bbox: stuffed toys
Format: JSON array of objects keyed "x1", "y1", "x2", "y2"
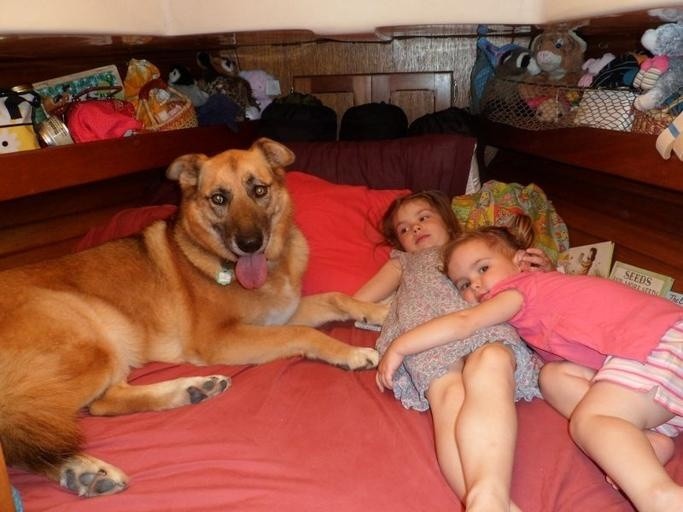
[{"x1": 479, "y1": 4, "x2": 683, "y2": 131}]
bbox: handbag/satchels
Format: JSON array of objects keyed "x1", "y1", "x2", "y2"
[
  {"x1": 0, "y1": 89, "x2": 42, "y2": 154},
  {"x1": 66, "y1": 95, "x2": 143, "y2": 143}
]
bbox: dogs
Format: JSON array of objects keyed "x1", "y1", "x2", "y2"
[{"x1": 0, "y1": 136, "x2": 394, "y2": 498}]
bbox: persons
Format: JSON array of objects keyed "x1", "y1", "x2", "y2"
[
  {"x1": 575, "y1": 248, "x2": 597, "y2": 275},
  {"x1": 372, "y1": 212, "x2": 683, "y2": 511},
  {"x1": 344, "y1": 187, "x2": 557, "y2": 510}
]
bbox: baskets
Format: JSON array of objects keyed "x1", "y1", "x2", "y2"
[{"x1": 133, "y1": 75, "x2": 198, "y2": 133}]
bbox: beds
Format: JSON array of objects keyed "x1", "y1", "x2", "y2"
[{"x1": 3, "y1": 134, "x2": 682, "y2": 512}]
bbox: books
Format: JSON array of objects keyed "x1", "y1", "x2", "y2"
[
  {"x1": 609, "y1": 260, "x2": 675, "y2": 300},
  {"x1": 564, "y1": 241, "x2": 614, "y2": 278}
]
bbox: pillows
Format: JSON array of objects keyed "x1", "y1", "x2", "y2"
[{"x1": 271, "y1": 169, "x2": 411, "y2": 257}]
